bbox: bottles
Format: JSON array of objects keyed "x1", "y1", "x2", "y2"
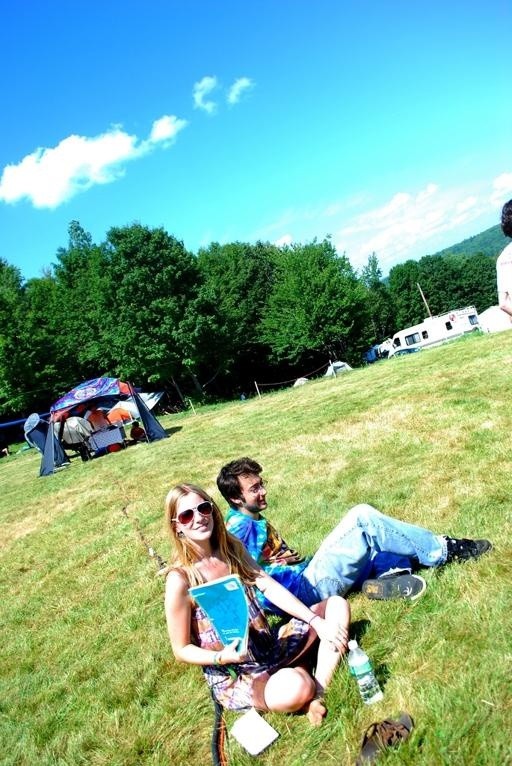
[{"x1": 345, "y1": 638, "x2": 384, "y2": 707}]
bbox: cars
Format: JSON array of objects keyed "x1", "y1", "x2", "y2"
[{"x1": 385, "y1": 347, "x2": 419, "y2": 360}]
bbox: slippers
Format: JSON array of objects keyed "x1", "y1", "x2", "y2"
[{"x1": 353, "y1": 712, "x2": 414, "y2": 766}]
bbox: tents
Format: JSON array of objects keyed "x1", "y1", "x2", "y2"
[
  {"x1": 322, "y1": 360, "x2": 355, "y2": 378},
  {"x1": 365, "y1": 344, "x2": 387, "y2": 363},
  {"x1": 23, "y1": 376, "x2": 170, "y2": 476},
  {"x1": 293, "y1": 377, "x2": 309, "y2": 388}
]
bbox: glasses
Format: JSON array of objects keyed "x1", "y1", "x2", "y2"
[
  {"x1": 241, "y1": 480, "x2": 268, "y2": 493},
  {"x1": 171, "y1": 501, "x2": 213, "y2": 526}
]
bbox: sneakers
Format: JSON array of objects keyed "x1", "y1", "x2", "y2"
[
  {"x1": 361, "y1": 573, "x2": 426, "y2": 601},
  {"x1": 438, "y1": 536, "x2": 491, "y2": 568}
]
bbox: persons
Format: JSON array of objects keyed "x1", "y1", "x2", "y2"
[
  {"x1": 494, "y1": 199, "x2": 512, "y2": 323},
  {"x1": 214, "y1": 456, "x2": 493, "y2": 615},
  {"x1": 130, "y1": 422, "x2": 146, "y2": 442},
  {"x1": 160, "y1": 483, "x2": 353, "y2": 729},
  {"x1": 86, "y1": 403, "x2": 113, "y2": 431}
]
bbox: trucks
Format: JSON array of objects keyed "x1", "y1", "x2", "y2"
[{"x1": 387, "y1": 303, "x2": 483, "y2": 361}]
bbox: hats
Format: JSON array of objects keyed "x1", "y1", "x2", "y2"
[{"x1": 281, "y1": 625, "x2": 318, "y2": 667}]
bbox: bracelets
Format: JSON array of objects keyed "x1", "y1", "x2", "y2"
[
  {"x1": 213, "y1": 652, "x2": 223, "y2": 670},
  {"x1": 307, "y1": 615, "x2": 319, "y2": 626}
]
